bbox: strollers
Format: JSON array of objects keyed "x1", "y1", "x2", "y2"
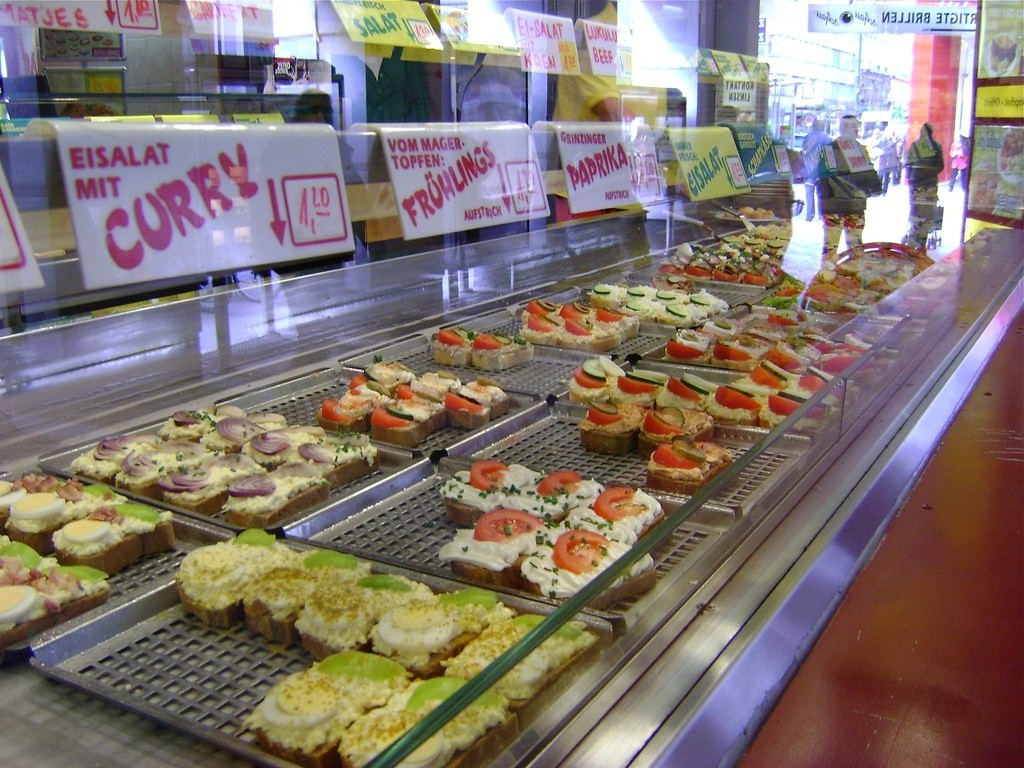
[{"x1": 901, "y1": 194, "x2": 944, "y2": 248}]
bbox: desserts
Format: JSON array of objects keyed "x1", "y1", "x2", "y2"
[{"x1": 0, "y1": 212, "x2": 935, "y2": 768}]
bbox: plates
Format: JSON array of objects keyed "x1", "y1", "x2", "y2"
[{"x1": 715, "y1": 214, "x2": 779, "y2": 225}]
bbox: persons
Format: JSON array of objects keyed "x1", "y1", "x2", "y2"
[
  {"x1": 255, "y1": 88, "x2": 386, "y2": 371},
  {"x1": 948, "y1": 134, "x2": 970, "y2": 192},
  {"x1": 907, "y1": 122, "x2": 945, "y2": 247},
  {"x1": 802, "y1": 117, "x2": 834, "y2": 222},
  {"x1": 817, "y1": 115, "x2": 882, "y2": 267},
  {"x1": 546, "y1": 0, "x2": 669, "y2": 292},
  {"x1": 864, "y1": 130, "x2": 905, "y2": 195}
]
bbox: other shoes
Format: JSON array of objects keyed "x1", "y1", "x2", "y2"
[{"x1": 806, "y1": 213, "x2": 814, "y2": 221}]
